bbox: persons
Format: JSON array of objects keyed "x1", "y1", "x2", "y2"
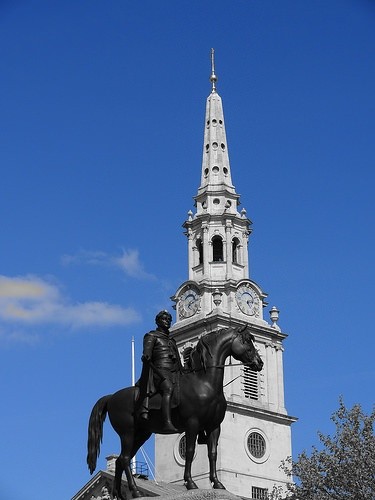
[{"x1": 136, "y1": 308, "x2": 183, "y2": 434}]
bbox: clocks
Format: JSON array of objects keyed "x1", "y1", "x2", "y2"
[
  {"x1": 178, "y1": 286, "x2": 199, "y2": 318},
  {"x1": 235, "y1": 285, "x2": 260, "y2": 315}
]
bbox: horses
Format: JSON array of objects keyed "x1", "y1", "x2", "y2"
[{"x1": 86, "y1": 322, "x2": 265, "y2": 500}]
